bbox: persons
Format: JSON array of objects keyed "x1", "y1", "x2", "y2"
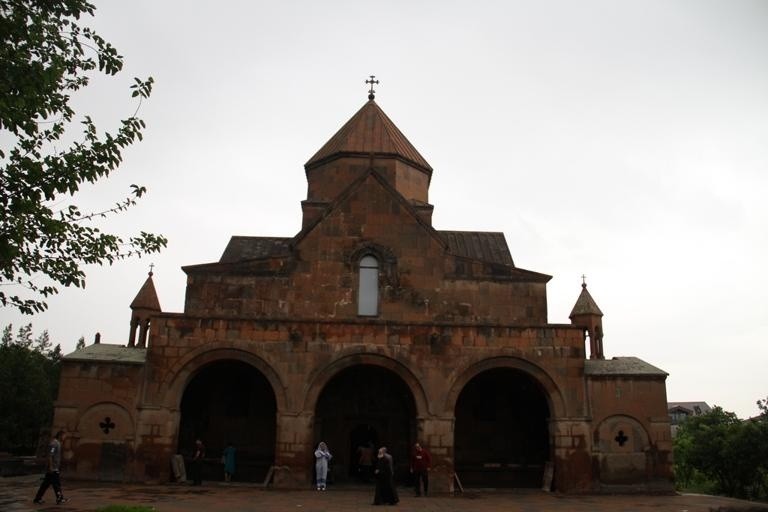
[
  {"x1": 381, "y1": 446, "x2": 399, "y2": 503},
  {"x1": 33, "y1": 430, "x2": 73, "y2": 505},
  {"x1": 188, "y1": 436, "x2": 207, "y2": 487},
  {"x1": 219, "y1": 442, "x2": 238, "y2": 482},
  {"x1": 370, "y1": 447, "x2": 395, "y2": 505},
  {"x1": 409, "y1": 441, "x2": 433, "y2": 498},
  {"x1": 314, "y1": 441, "x2": 334, "y2": 491},
  {"x1": 359, "y1": 441, "x2": 374, "y2": 476}
]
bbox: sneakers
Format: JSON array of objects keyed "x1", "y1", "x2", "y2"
[
  {"x1": 317, "y1": 486, "x2": 326, "y2": 492},
  {"x1": 56, "y1": 495, "x2": 72, "y2": 504},
  {"x1": 33, "y1": 499, "x2": 45, "y2": 504}
]
